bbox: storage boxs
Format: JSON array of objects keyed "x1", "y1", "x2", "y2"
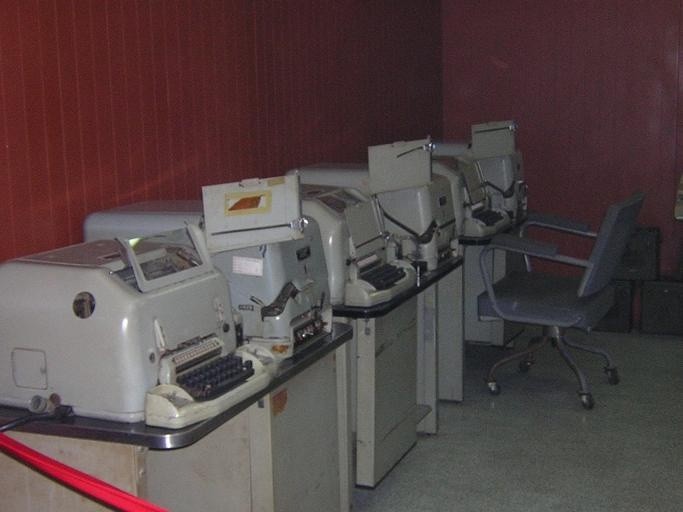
[
  {"x1": 593, "y1": 278, "x2": 632, "y2": 332},
  {"x1": 640, "y1": 275, "x2": 683, "y2": 336},
  {"x1": 610, "y1": 226, "x2": 660, "y2": 281}
]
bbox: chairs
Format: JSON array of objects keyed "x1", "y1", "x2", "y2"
[{"x1": 476, "y1": 192, "x2": 644, "y2": 410}]
